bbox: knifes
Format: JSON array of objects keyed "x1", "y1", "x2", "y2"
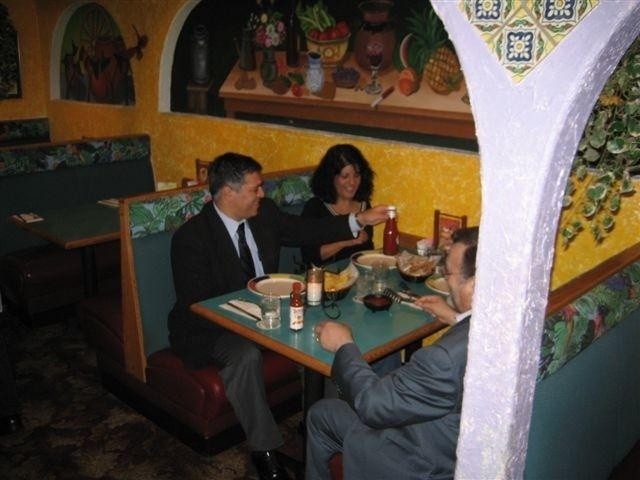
[
  {"x1": 371, "y1": 87, "x2": 395, "y2": 109},
  {"x1": 227, "y1": 302, "x2": 262, "y2": 323}
]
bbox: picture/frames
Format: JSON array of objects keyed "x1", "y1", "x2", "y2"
[{"x1": 1, "y1": 30, "x2": 23, "y2": 101}]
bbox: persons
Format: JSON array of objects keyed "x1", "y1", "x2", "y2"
[
  {"x1": 166, "y1": 152, "x2": 405, "y2": 478},
  {"x1": 306, "y1": 225, "x2": 478, "y2": 479},
  {"x1": 300, "y1": 143, "x2": 401, "y2": 376}
]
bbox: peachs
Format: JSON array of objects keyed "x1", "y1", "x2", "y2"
[{"x1": 398, "y1": 66, "x2": 421, "y2": 97}]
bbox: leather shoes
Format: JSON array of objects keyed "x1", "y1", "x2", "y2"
[
  {"x1": 251, "y1": 450, "x2": 289, "y2": 480},
  {"x1": 1, "y1": 415, "x2": 22, "y2": 436}
]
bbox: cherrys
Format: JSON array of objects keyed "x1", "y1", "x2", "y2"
[
  {"x1": 292, "y1": 83, "x2": 305, "y2": 98},
  {"x1": 233, "y1": 69, "x2": 257, "y2": 90}
]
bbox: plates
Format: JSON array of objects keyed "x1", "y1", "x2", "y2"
[
  {"x1": 349, "y1": 250, "x2": 398, "y2": 272},
  {"x1": 425, "y1": 273, "x2": 453, "y2": 296},
  {"x1": 247, "y1": 272, "x2": 310, "y2": 298}
]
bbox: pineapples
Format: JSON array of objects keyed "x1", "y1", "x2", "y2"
[{"x1": 403, "y1": 7, "x2": 464, "y2": 96}]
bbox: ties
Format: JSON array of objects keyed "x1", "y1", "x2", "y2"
[{"x1": 237, "y1": 223, "x2": 255, "y2": 278}]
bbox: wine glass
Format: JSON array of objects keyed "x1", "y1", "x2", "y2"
[{"x1": 363, "y1": 43, "x2": 384, "y2": 95}]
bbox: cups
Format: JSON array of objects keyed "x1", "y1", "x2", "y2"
[
  {"x1": 371, "y1": 261, "x2": 389, "y2": 294},
  {"x1": 356, "y1": 270, "x2": 377, "y2": 302},
  {"x1": 260, "y1": 294, "x2": 281, "y2": 329}
]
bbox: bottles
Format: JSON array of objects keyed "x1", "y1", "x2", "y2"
[
  {"x1": 288, "y1": 282, "x2": 304, "y2": 331},
  {"x1": 382, "y1": 205, "x2": 399, "y2": 255},
  {"x1": 286, "y1": 7, "x2": 301, "y2": 68}
]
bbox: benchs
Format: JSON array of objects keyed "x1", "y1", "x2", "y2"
[
  {"x1": 1, "y1": 118, "x2": 53, "y2": 145},
  {"x1": 118, "y1": 163, "x2": 419, "y2": 457},
  {"x1": 398, "y1": 244, "x2": 637, "y2": 480},
  {"x1": 2, "y1": 134, "x2": 156, "y2": 305}
]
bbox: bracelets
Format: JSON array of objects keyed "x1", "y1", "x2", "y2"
[{"x1": 354, "y1": 213, "x2": 365, "y2": 229}]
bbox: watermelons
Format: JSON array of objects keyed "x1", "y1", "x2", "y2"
[{"x1": 393, "y1": 31, "x2": 423, "y2": 81}]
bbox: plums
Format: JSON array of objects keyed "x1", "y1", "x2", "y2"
[{"x1": 271, "y1": 73, "x2": 291, "y2": 95}]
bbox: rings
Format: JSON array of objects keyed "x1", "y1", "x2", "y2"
[{"x1": 316, "y1": 336, "x2": 320, "y2": 342}]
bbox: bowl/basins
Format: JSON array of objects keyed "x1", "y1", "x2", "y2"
[{"x1": 399, "y1": 265, "x2": 436, "y2": 283}]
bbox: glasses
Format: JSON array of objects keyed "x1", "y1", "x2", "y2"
[{"x1": 438, "y1": 269, "x2": 454, "y2": 278}]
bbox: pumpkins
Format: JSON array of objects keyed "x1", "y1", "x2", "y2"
[{"x1": 88, "y1": 30, "x2": 127, "y2": 102}]
